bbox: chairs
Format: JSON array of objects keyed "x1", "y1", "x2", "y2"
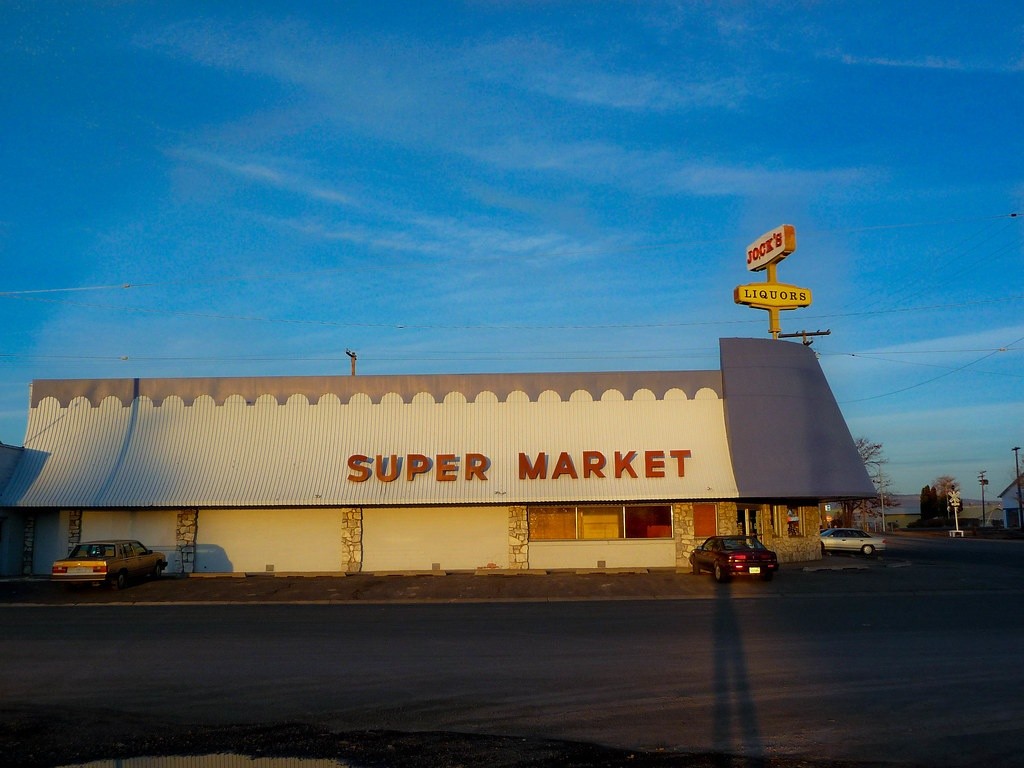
[
  {"x1": 77, "y1": 550, "x2": 88, "y2": 558},
  {"x1": 105, "y1": 550, "x2": 114, "y2": 556}
]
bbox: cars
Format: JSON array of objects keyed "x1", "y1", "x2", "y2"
[
  {"x1": 50, "y1": 539, "x2": 168, "y2": 590},
  {"x1": 688, "y1": 535, "x2": 780, "y2": 583},
  {"x1": 819, "y1": 528, "x2": 887, "y2": 554}
]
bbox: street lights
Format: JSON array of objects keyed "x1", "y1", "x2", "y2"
[{"x1": 1011, "y1": 446, "x2": 1024, "y2": 531}]
